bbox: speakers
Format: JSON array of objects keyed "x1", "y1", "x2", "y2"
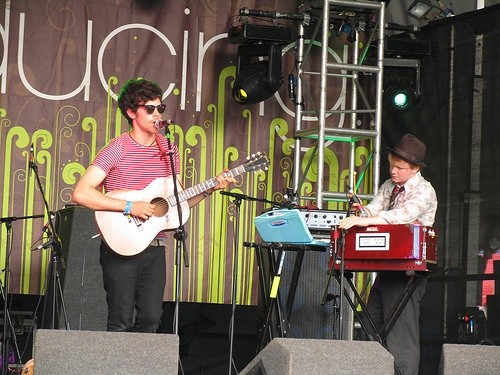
[
  {"x1": 438, "y1": 343, "x2": 500, "y2": 375},
  {"x1": 273, "y1": 243, "x2": 355, "y2": 340},
  {"x1": 44, "y1": 206, "x2": 109, "y2": 331},
  {"x1": 33, "y1": 329, "x2": 179, "y2": 375},
  {"x1": 238, "y1": 338, "x2": 394, "y2": 375}
]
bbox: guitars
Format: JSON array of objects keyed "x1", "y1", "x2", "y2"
[{"x1": 94, "y1": 151, "x2": 270, "y2": 257}]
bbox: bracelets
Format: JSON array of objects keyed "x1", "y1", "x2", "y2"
[{"x1": 123, "y1": 200, "x2": 132, "y2": 215}]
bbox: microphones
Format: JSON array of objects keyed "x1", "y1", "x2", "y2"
[
  {"x1": 37, "y1": 212, "x2": 56, "y2": 240},
  {"x1": 155, "y1": 119, "x2": 174, "y2": 130},
  {"x1": 29, "y1": 143, "x2": 34, "y2": 168},
  {"x1": 347, "y1": 185, "x2": 368, "y2": 218},
  {"x1": 38, "y1": 238, "x2": 55, "y2": 250},
  {"x1": 326, "y1": 294, "x2": 340, "y2": 301}
]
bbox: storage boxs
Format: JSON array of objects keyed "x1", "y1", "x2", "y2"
[{"x1": 331, "y1": 224, "x2": 438, "y2": 264}]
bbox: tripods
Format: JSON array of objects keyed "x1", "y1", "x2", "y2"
[{"x1": 32, "y1": 165, "x2": 70, "y2": 329}]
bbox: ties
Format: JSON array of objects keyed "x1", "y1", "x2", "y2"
[{"x1": 388, "y1": 185, "x2": 405, "y2": 210}]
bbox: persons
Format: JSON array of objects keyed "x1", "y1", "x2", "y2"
[
  {"x1": 338, "y1": 133, "x2": 437, "y2": 375},
  {"x1": 72, "y1": 79, "x2": 232, "y2": 333}
]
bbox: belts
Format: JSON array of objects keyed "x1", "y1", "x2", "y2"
[{"x1": 150, "y1": 240, "x2": 168, "y2": 248}]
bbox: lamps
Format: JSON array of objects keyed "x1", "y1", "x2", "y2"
[
  {"x1": 232, "y1": 58, "x2": 282, "y2": 105},
  {"x1": 407, "y1": 0, "x2": 432, "y2": 22},
  {"x1": 382, "y1": 78, "x2": 414, "y2": 113}
]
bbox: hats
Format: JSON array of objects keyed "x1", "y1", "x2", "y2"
[{"x1": 385, "y1": 134, "x2": 428, "y2": 168}]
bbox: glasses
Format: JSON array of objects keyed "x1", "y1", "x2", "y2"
[{"x1": 137, "y1": 104, "x2": 166, "y2": 114}]
